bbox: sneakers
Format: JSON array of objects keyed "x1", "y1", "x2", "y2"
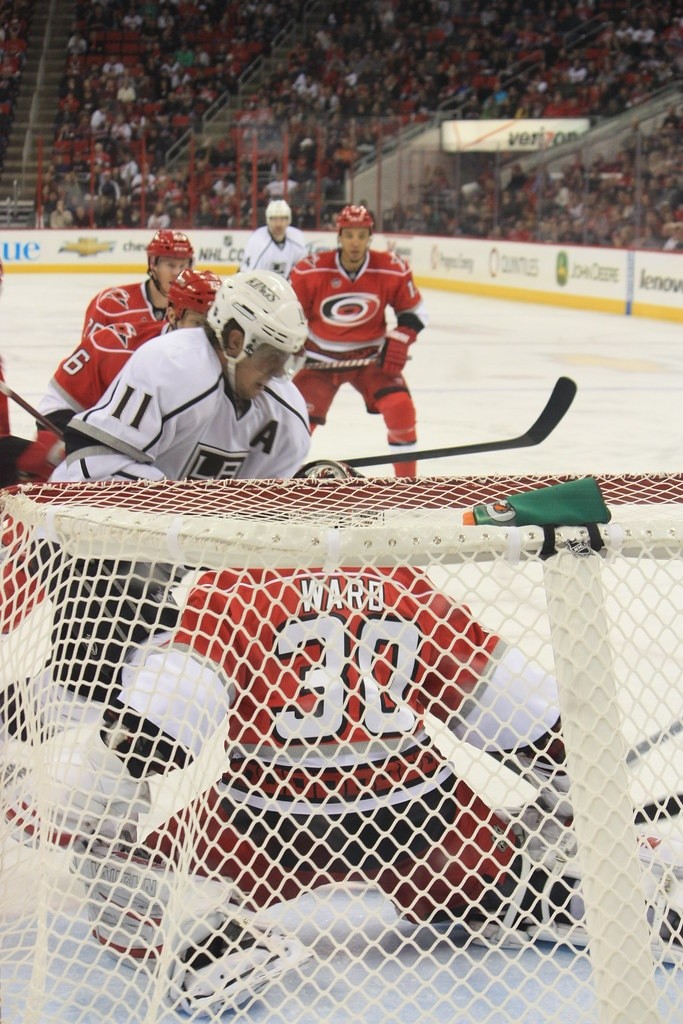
[{"x1": 179, "y1": 925, "x2": 312, "y2": 1015}]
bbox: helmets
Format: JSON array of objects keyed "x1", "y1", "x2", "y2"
[
  {"x1": 264, "y1": 200, "x2": 292, "y2": 227},
  {"x1": 144, "y1": 230, "x2": 193, "y2": 270},
  {"x1": 206, "y1": 268, "x2": 310, "y2": 357},
  {"x1": 161, "y1": 269, "x2": 223, "y2": 320},
  {"x1": 336, "y1": 204, "x2": 375, "y2": 235}
]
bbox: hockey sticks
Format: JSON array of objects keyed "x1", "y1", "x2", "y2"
[
  {"x1": 333, "y1": 374, "x2": 578, "y2": 468},
  {"x1": 292, "y1": 353, "x2": 414, "y2": 370}
]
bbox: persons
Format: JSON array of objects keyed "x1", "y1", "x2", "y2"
[
  {"x1": 0, "y1": 0, "x2": 683, "y2": 252},
  {"x1": 238, "y1": 199, "x2": 309, "y2": 279},
  {"x1": 82, "y1": 230, "x2": 194, "y2": 341},
  {"x1": 0, "y1": 269, "x2": 312, "y2": 766},
  {"x1": 1, "y1": 269, "x2": 222, "y2": 634},
  {"x1": 1, "y1": 459, "x2": 682, "y2": 1018},
  {"x1": 287, "y1": 204, "x2": 426, "y2": 478}
]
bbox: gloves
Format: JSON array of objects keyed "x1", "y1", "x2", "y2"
[{"x1": 377, "y1": 326, "x2": 416, "y2": 377}]
